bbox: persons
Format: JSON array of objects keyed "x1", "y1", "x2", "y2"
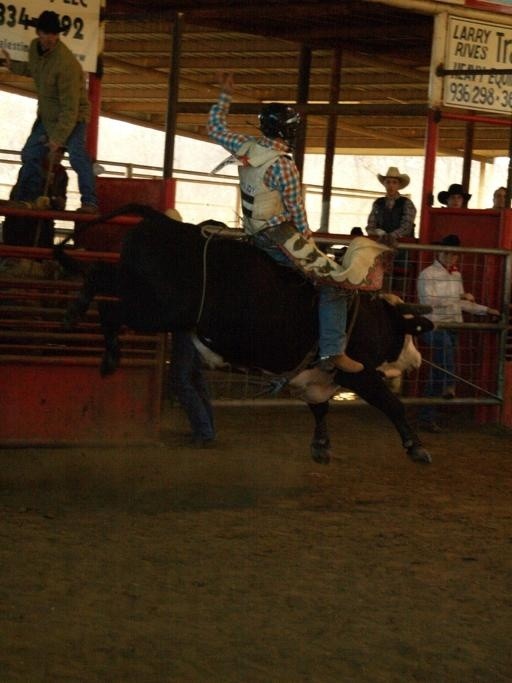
[
  {"x1": 489, "y1": 186, "x2": 506, "y2": 207},
  {"x1": 2, "y1": 6, "x2": 101, "y2": 214},
  {"x1": 437, "y1": 182, "x2": 471, "y2": 207},
  {"x1": 2, "y1": 139, "x2": 71, "y2": 248},
  {"x1": 202, "y1": 69, "x2": 366, "y2": 373},
  {"x1": 368, "y1": 166, "x2": 417, "y2": 298},
  {"x1": 416, "y1": 233, "x2": 500, "y2": 435}
]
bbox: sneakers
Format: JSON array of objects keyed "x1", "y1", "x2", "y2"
[
  {"x1": 441, "y1": 384, "x2": 456, "y2": 398},
  {"x1": 416, "y1": 421, "x2": 449, "y2": 434},
  {"x1": 321, "y1": 352, "x2": 365, "y2": 373},
  {"x1": 78, "y1": 203, "x2": 100, "y2": 215}
]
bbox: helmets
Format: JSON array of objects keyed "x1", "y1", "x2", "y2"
[{"x1": 257, "y1": 103, "x2": 303, "y2": 150}]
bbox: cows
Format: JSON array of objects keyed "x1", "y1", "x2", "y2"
[{"x1": 51, "y1": 200, "x2": 436, "y2": 470}]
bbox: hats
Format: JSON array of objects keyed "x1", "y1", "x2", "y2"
[
  {"x1": 19, "y1": 11, "x2": 70, "y2": 34},
  {"x1": 437, "y1": 184, "x2": 471, "y2": 204},
  {"x1": 430, "y1": 234, "x2": 460, "y2": 246},
  {"x1": 377, "y1": 167, "x2": 410, "y2": 189}
]
